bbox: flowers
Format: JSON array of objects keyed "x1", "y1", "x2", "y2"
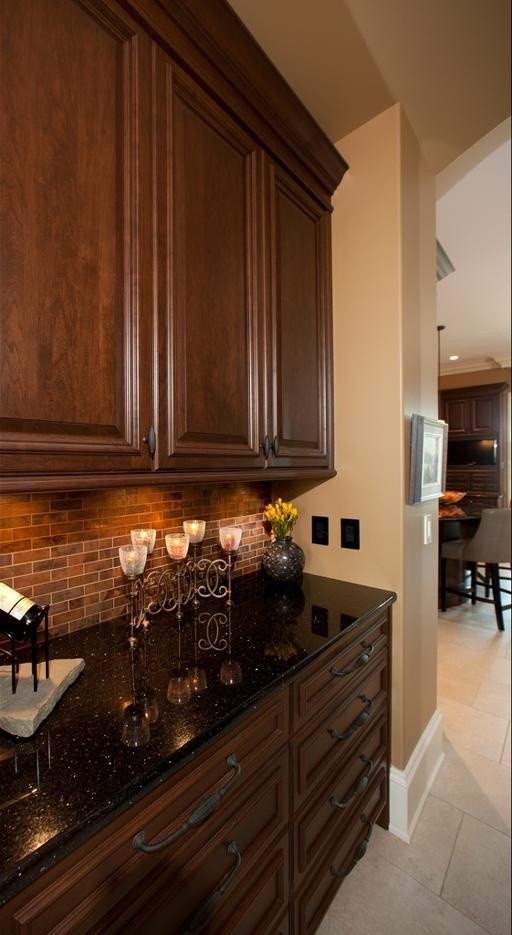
[{"x1": 260, "y1": 497, "x2": 301, "y2": 537}]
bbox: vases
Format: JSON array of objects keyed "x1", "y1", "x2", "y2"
[{"x1": 260, "y1": 536, "x2": 310, "y2": 583}]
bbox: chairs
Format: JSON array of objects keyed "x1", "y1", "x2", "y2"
[{"x1": 439, "y1": 503, "x2": 511, "y2": 633}]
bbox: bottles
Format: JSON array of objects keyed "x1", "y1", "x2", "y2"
[{"x1": 0, "y1": 580, "x2": 45, "y2": 637}]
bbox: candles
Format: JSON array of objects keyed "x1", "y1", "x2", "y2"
[{"x1": 111, "y1": 518, "x2": 246, "y2": 580}]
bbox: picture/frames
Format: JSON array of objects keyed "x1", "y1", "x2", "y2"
[{"x1": 408, "y1": 413, "x2": 452, "y2": 506}]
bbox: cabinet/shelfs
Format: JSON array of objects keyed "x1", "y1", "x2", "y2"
[
  {"x1": 149, "y1": 1, "x2": 352, "y2": 490},
  {"x1": 1, "y1": 2, "x2": 148, "y2": 496},
  {"x1": 3, "y1": 567, "x2": 397, "y2": 934},
  {"x1": 439, "y1": 381, "x2": 510, "y2": 495}
]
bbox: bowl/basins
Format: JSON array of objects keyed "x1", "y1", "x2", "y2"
[
  {"x1": 440, "y1": 490, "x2": 466, "y2": 505},
  {"x1": 438, "y1": 505, "x2": 467, "y2": 520}
]
